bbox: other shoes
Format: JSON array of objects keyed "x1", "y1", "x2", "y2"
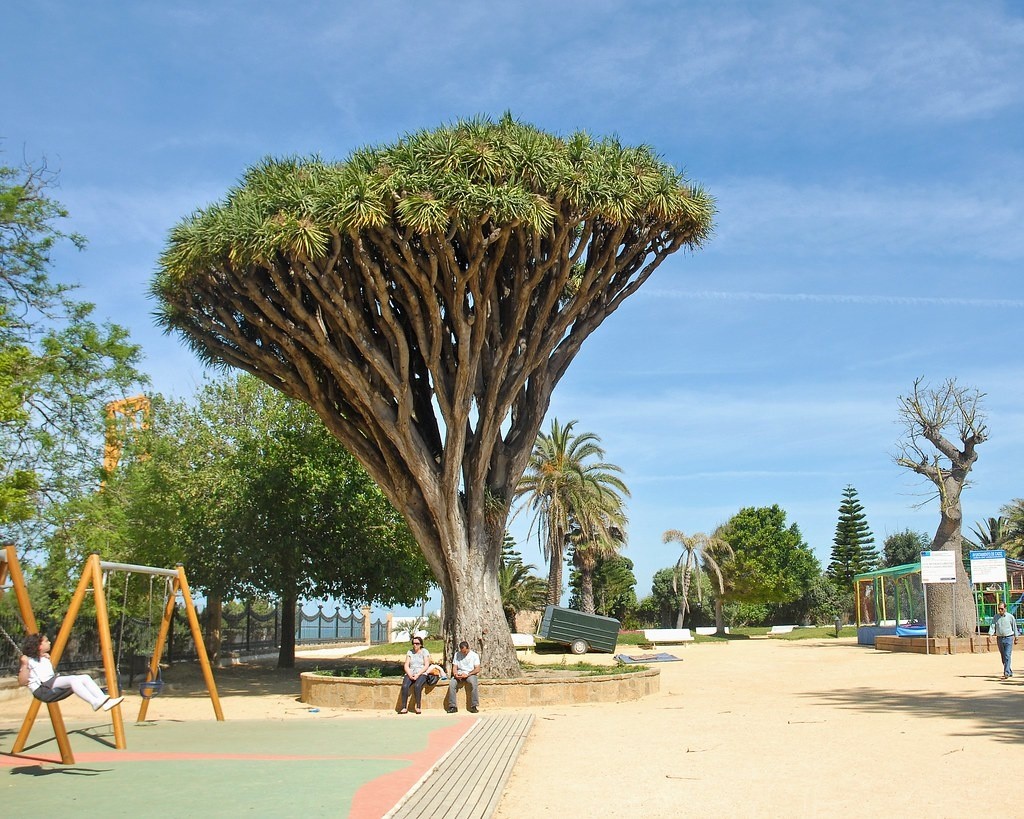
[
  {"x1": 400, "y1": 709, "x2": 408, "y2": 713},
  {"x1": 415, "y1": 708, "x2": 421, "y2": 714}
]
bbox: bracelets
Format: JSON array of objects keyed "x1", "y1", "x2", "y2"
[
  {"x1": 19, "y1": 668, "x2": 30, "y2": 672},
  {"x1": 1015, "y1": 638, "x2": 1019, "y2": 640}
]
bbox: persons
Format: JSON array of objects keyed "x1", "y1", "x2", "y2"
[
  {"x1": 17, "y1": 632, "x2": 125, "y2": 711},
  {"x1": 400, "y1": 637, "x2": 429, "y2": 714},
  {"x1": 988, "y1": 602, "x2": 1018, "y2": 679},
  {"x1": 447, "y1": 641, "x2": 480, "y2": 713}
]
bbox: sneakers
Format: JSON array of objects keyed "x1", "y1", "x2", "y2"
[
  {"x1": 91, "y1": 695, "x2": 111, "y2": 712},
  {"x1": 471, "y1": 707, "x2": 478, "y2": 713},
  {"x1": 447, "y1": 707, "x2": 458, "y2": 713},
  {"x1": 102, "y1": 695, "x2": 125, "y2": 711}
]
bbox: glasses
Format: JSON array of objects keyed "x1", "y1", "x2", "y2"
[{"x1": 412, "y1": 643, "x2": 420, "y2": 646}]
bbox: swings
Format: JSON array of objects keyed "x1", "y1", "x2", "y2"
[
  {"x1": 99, "y1": 573, "x2": 129, "y2": 699},
  {"x1": 139, "y1": 577, "x2": 168, "y2": 700},
  {"x1": 0, "y1": 590, "x2": 74, "y2": 704}
]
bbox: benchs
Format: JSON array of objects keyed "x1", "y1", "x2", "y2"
[
  {"x1": 767, "y1": 626, "x2": 795, "y2": 635},
  {"x1": 510, "y1": 633, "x2": 537, "y2": 655},
  {"x1": 696, "y1": 627, "x2": 729, "y2": 635},
  {"x1": 644, "y1": 628, "x2": 695, "y2": 649}
]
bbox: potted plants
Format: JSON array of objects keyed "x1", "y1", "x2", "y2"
[{"x1": 134, "y1": 649, "x2": 149, "y2": 673}]
